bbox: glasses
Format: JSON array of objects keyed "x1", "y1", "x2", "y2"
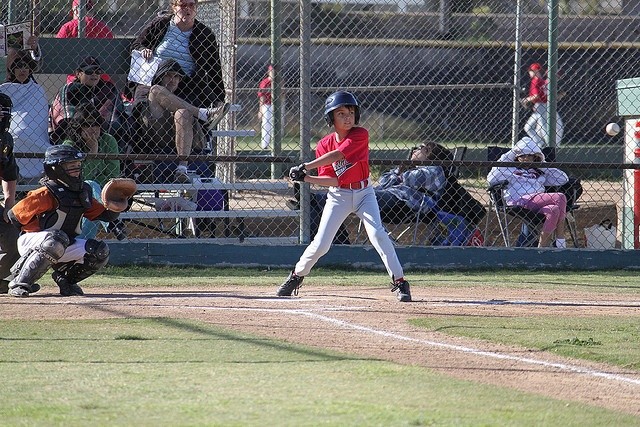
[
  {"x1": 16, "y1": 62, "x2": 30, "y2": 69},
  {"x1": 83, "y1": 120, "x2": 101, "y2": 126},
  {"x1": 416, "y1": 145, "x2": 430, "y2": 151},
  {"x1": 82, "y1": 69, "x2": 103, "y2": 75},
  {"x1": 179, "y1": 2, "x2": 196, "y2": 8}
]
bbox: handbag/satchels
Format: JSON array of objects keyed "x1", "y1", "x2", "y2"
[{"x1": 584, "y1": 218, "x2": 617, "y2": 248}]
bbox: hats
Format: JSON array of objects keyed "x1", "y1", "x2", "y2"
[
  {"x1": 154, "y1": 58, "x2": 186, "y2": 84},
  {"x1": 529, "y1": 63, "x2": 541, "y2": 71},
  {"x1": 68, "y1": 0, "x2": 93, "y2": 16},
  {"x1": 6, "y1": 49, "x2": 38, "y2": 72},
  {"x1": 77, "y1": 55, "x2": 102, "y2": 70}
]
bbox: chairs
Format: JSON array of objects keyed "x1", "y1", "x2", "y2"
[
  {"x1": 352, "y1": 146, "x2": 467, "y2": 246},
  {"x1": 483, "y1": 146, "x2": 582, "y2": 247}
]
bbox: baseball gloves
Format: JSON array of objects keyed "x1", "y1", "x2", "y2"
[{"x1": 101, "y1": 177, "x2": 136, "y2": 213}]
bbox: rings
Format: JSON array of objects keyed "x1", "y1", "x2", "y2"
[{"x1": 33, "y1": 41, "x2": 36, "y2": 44}]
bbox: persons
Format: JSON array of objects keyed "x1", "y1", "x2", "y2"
[
  {"x1": 407, "y1": 147, "x2": 487, "y2": 246},
  {"x1": 59, "y1": 104, "x2": 127, "y2": 244},
  {"x1": 7, "y1": 145, "x2": 137, "y2": 298},
  {"x1": 258, "y1": 63, "x2": 274, "y2": 149},
  {"x1": 0, "y1": 44, "x2": 53, "y2": 196},
  {"x1": 125, "y1": 0, "x2": 226, "y2": 140},
  {"x1": 485, "y1": 136, "x2": 570, "y2": 248},
  {"x1": 0, "y1": 91, "x2": 40, "y2": 295},
  {"x1": 520, "y1": 63, "x2": 548, "y2": 149},
  {"x1": 132, "y1": 60, "x2": 231, "y2": 183},
  {"x1": 285, "y1": 140, "x2": 454, "y2": 225},
  {"x1": 55, "y1": 0, "x2": 116, "y2": 84},
  {"x1": 48, "y1": 55, "x2": 131, "y2": 149},
  {"x1": 0, "y1": 24, "x2": 43, "y2": 61},
  {"x1": 276, "y1": 90, "x2": 413, "y2": 302},
  {"x1": 542, "y1": 65, "x2": 568, "y2": 149}
]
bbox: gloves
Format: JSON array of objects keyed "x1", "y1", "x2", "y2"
[
  {"x1": 290, "y1": 166, "x2": 306, "y2": 182},
  {"x1": 298, "y1": 162, "x2": 308, "y2": 174}
]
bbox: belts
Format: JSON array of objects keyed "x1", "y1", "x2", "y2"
[{"x1": 336, "y1": 180, "x2": 368, "y2": 190}]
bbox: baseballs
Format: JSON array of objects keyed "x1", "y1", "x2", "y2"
[{"x1": 606, "y1": 122, "x2": 620, "y2": 136}]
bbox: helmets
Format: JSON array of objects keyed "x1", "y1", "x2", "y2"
[
  {"x1": 324, "y1": 90, "x2": 360, "y2": 126},
  {"x1": 1, "y1": 91, "x2": 13, "y2": 131},
  {"x1": 43, "y1": 144, "x2": 86, "y2": 191}
]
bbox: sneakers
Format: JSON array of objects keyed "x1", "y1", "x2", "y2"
[
  {"x1": 111, "y1": 221, "x2": 127, "y2": 240},
  {"x1": 0, "y1": 279, "x2": 40, "y2": 293},
  {"x1": 52, "y1": 270, "x2": 84, "y2": 295},
  {"x1": 8, "y1": 280, "x2": 29, "y2": 297},
  {"x1": 388, "y1": 274, "x2": 412, "y2": 301},
  {"x1": 277, "y1": 270, "x2": 303, "y2": 295},
  {"x1": 198, "y1": 102, "x2": 230, "y2": 131},
  {"x1": 174, "y1": 171, "x2": 190, "y2": 183}
]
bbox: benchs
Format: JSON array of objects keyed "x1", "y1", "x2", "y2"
[{"x1": 1, "y1": 38, "x2": 298, "y2": 238}]
